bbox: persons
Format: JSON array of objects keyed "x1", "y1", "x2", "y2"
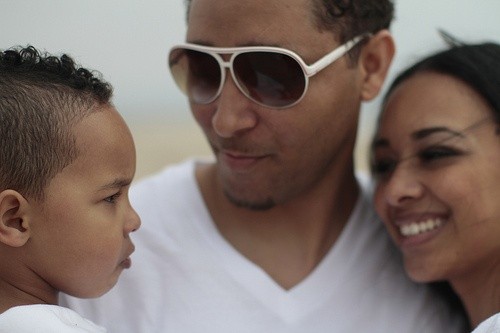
[
  {"x1": 53, "y1": 0, "x2": 467, "y2": 332},
  {"x1": 367, "y1": 39, "x2": 500, "y2": 333},
  {"x1": 0, "y1": 41, "x2": 140, "y2": 333}
]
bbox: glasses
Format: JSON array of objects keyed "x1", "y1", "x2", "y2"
[{"x1": 167, "y1": 33, "x2": 374, "y2": 110}]
